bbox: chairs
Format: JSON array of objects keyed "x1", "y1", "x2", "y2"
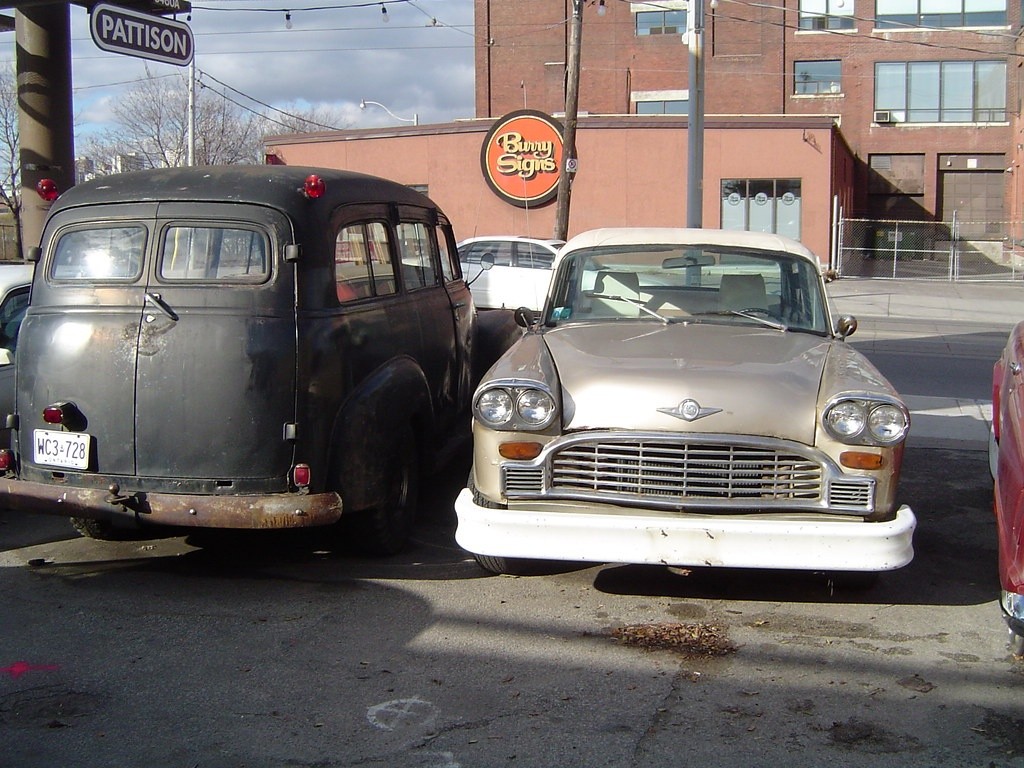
[
  {"x1": 719, "y1": 273, "x2": 768, "y2": 314},
  {"x1": 594, "y1": 271, "x2": 639, "y2": 318}
]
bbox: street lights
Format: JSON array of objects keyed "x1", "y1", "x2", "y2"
[{"x1": 359, "y1": 99, "x2": 419, "y2": 126}]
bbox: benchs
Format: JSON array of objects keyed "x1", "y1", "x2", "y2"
[{"x1": 577, "y1": 289, "x2": 781, "y2": 318}]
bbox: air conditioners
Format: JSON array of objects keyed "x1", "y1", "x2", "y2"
[{"x1": 873, "y1": 111, "x2": 890, "y2": 122}]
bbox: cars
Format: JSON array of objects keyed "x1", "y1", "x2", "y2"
[
  {"x1": 0, "y1": 164, "x2": 524, "y2": 552},
  {"x1": 453, "y1": 227, "x2": 917, "y2": 591},
  {"x1": 393, "y1": 236, "x2": 642, "y2": 325}
]
